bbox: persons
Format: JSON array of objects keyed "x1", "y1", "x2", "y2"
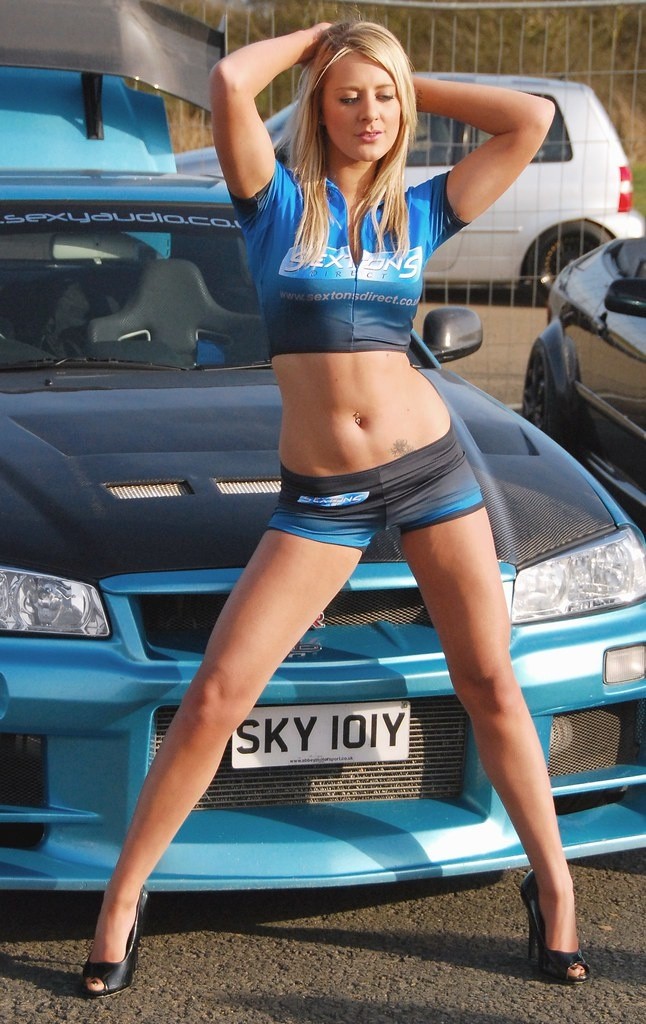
[{"x1": 82, "y1": 20, "x2": 590, "y2": 997}]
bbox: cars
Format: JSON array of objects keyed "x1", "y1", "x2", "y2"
[{"x1": 0, "y1": 0, "x2": 646, "y2": 892}]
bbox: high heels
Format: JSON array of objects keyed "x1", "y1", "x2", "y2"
[
  {"x1": 81, "y1": 886, "x2": 147, "y2": 999},
  {"x1": 519, "y1": 875, "x2": 592, "y2": 981}
]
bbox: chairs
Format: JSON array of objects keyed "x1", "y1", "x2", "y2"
[{"x1": 85, "y1": 258, "x2": 267, "y2": 371}]
